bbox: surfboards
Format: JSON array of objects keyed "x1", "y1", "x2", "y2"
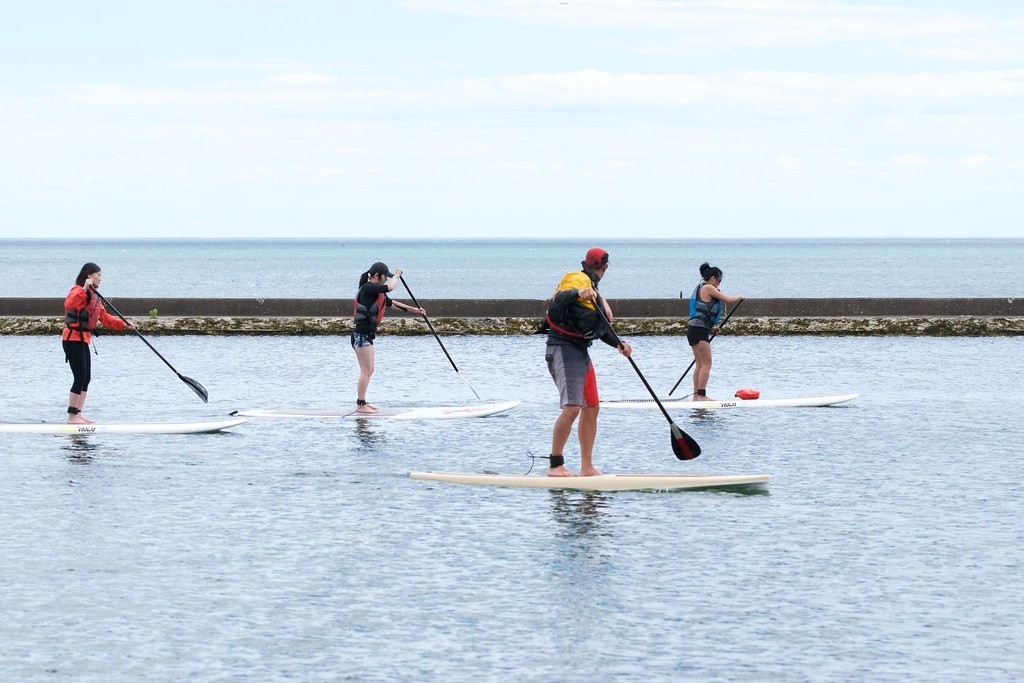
[
  {"x1": 0, "y1": 416, "x2": 248, "y2": 435},
  {"x1": 598, "y1": 394, "x2": 860, "y2": 409},
  {"x1": 409, "y1": 470, "x2": 772, "y2": 495},
  {"x1": 239, "y1": 399, "x2": 522, "y2": 418}
]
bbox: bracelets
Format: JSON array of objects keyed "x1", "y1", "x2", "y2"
[{"x1": 393, "y1": 274, "x2": 401, "y2": 281}]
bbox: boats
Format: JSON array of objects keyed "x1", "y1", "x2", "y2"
[
  {"x1": 409, "y1": 470, "x2": 773, "y2": 493},
  {"x1": 0, "y1": 418, "x2": 247, "y2": 435},
  {"x1": 236, "y1": 399, "x2": 523, "y2": 419},
  {"x1": 599, "y1": 392, "x2": 860, "y2": 408}
]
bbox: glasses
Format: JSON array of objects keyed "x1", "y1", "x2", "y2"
[{"x1": 717, "y1": 278, "x2": 721, "y2": 283}]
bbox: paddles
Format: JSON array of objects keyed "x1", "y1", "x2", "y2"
[
  {"x1": 667, "y1": 297, "x2": 744, "y2": 399},
  {"x1": 398, "y1": 275, "x2": 482, "y2": 402},
  {"x1": 589, "y1": 297, "x2": 702, "y2": 461},
  {"x1": 87, "y1": 285, "x2": 209, "y2": 404}
]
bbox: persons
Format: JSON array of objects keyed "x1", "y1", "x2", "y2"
[
  {"x1": 544, "y1": 247, "x2": 633, "y2": 478},
  {"x1": 685, "y1": 260, "x2": 747, "y2": 402},
  {"x1": 60, "y1": 262, "x2": 138, "y2": 425},
  {"x1": 350, "y1": 261, "x2": 427, "y2": 413}
]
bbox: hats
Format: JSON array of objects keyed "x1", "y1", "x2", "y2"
[
  {"x1": 370, "y1": 262, "x2": 395, "y2": 278},
  {"x1": 585, "y1": 248, "x2": 609, "y2": 268}
]
